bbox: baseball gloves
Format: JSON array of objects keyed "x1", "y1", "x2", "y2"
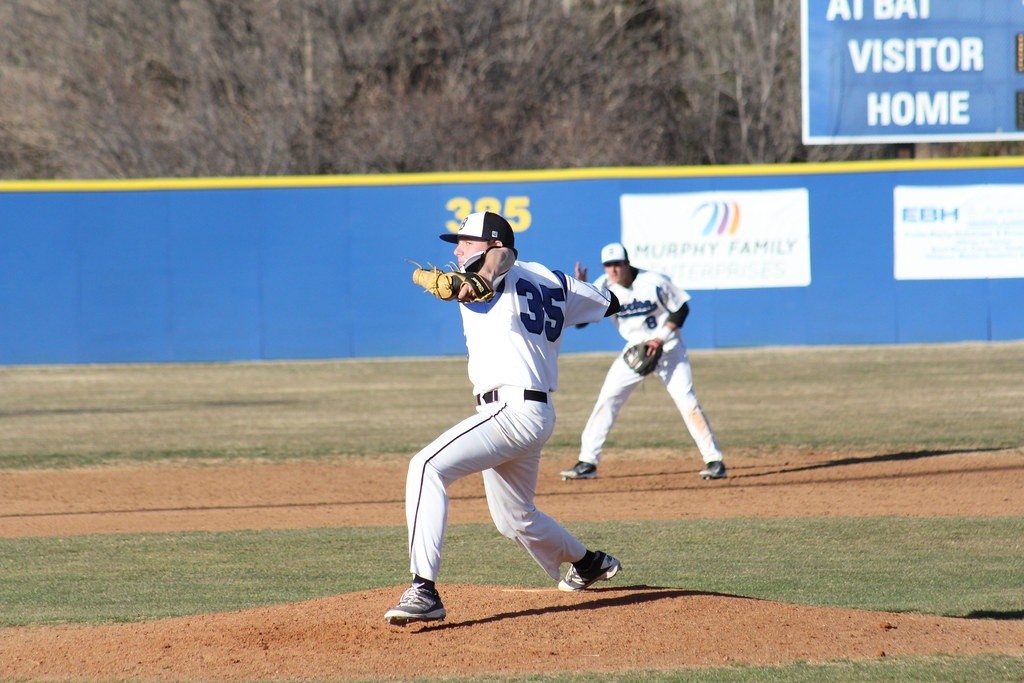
[
  {"x1": 623, "y1": 341, "x2": 663, "y2": 377},
  {"x1": 412, "y1": 258, "x2": 498, "y2": 304}
]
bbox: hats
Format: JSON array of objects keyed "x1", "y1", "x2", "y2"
[
  {"x1": 438, "y1": 212, "x2": 515, "y2": 250},
  {"x1": 601, "y1": 241, "x2": 628, "y2": 264}
]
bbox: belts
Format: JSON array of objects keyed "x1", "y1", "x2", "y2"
[{"x1": 476, "y1": 389, "x2": 547, "y2": 405}]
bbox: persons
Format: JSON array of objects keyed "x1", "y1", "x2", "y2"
[
  {"x1": 559, "y1": 243, "x2": 727, "y2": 482},
  {"x1": 382, "y1": 211, "x2": 621, "y2": 622}
]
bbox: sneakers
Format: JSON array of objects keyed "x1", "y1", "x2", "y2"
[
  {"x1": 560, "y1": 462, "x2": 596, "y2": 479},
  {"x1": 558, "y1": 550, "x2": 622, "y2": 591},
  {"x1": 699, "y1": 461, "x2": 727, "y2": 478},
  {"x1": 384, "y1": 582, "x2": 446, "y2": 627}
]
